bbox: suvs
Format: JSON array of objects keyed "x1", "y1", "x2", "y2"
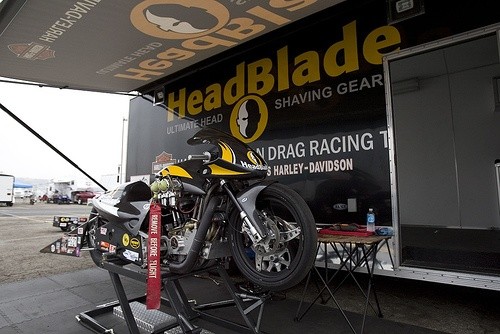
[{"x1": 70, "y1": 190, "x2": 96, "y2": 204}]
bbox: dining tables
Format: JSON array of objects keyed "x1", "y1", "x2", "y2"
[{"x1": 295, "y1": 224, "x2": 395, "y2": 334}]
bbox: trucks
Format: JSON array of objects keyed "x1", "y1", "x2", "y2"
[
  {"x1": 0, "y1": 0, "x2": 500, "y2": 291},
  {"x1": 50, "y1": 180, "x2": 73, "y2": 204},
  {"x1": 0, "y1": 173, "x2": 15, "y2": 207}
]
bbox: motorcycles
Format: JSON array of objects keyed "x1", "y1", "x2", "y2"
[{"x1": 85, "y1": 125, "x2": 317, "y2": 292}]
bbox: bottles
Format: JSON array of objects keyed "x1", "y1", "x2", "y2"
[{"x1": 366, "y1": 208, "x2": 376, "y2": 232}]
